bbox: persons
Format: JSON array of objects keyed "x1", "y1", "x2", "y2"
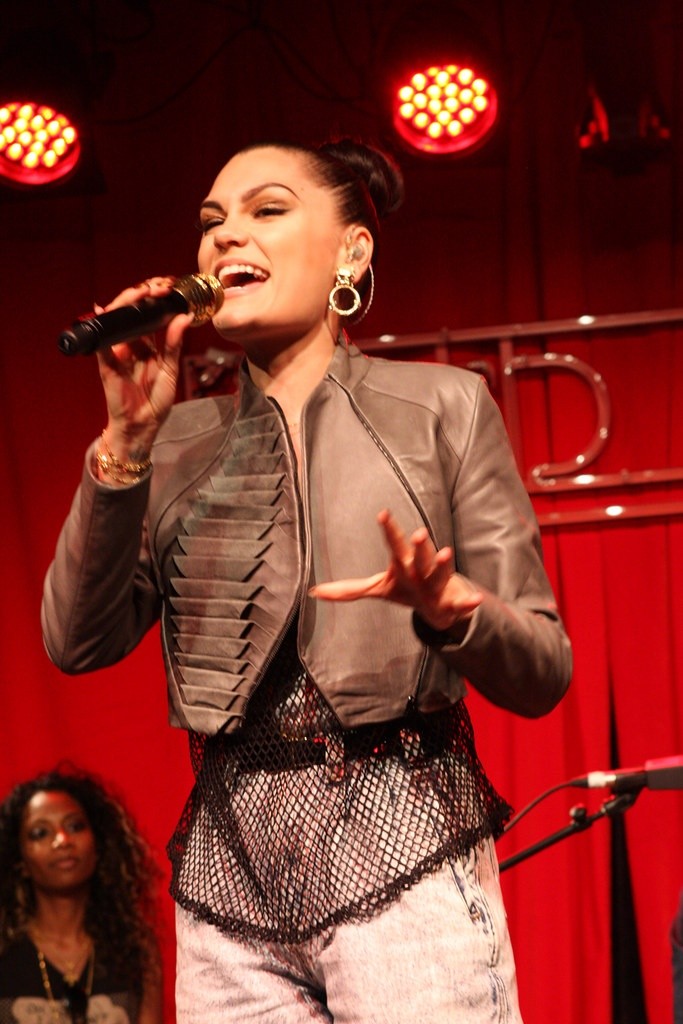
[
  {"x1": 0, "y1": 759, "x2": 162, "y2": 1024},
  {"x1": 41, "y1": 138, "x2": 573, "y2": 1024}
]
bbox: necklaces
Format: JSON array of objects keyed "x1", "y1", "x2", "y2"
[
  {"x1": 288, "y1": 423, "x2": 300, "y2": 436},
  {"x1": 39, "y1": 939, "x2": 95, "y2": 1022}
]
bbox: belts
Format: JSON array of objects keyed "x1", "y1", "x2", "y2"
[{"x1": 200, "y1": 701, "x2": 467, "y2": 777}]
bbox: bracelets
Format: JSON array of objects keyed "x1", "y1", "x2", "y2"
[{"x1": 96, "y1": 430, "x2": 151, "y2": 485}]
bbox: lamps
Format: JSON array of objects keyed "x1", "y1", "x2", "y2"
[
  {"x1": 370, "y1": 0, "x2": 506, "y2": 167},
  {"x1": 0, "y1": 16, "x2": 117, "y2": 199}
]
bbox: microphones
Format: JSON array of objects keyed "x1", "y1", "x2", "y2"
[
  {"x1": 56, "y1": 275, "x2": 225, "y2": 355},
  {"x1": 570, "y1": 766, "x2": 647, "y2": 792}
]
